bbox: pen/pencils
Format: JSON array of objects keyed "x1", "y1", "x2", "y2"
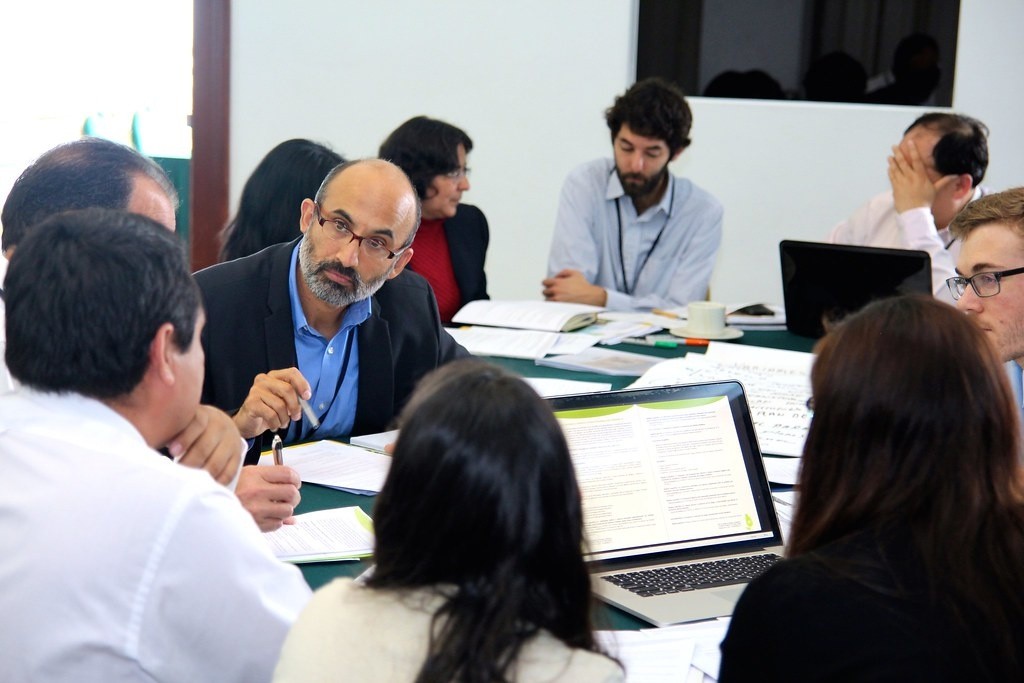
[
  {"x1": 271, "y1": 434, "x2": 283, "y2": 467},
  {"x1": 649, "y1": 309, "x2": 687, "y2": 320},
  {"x1": 623, "y1": 340, "x2": 679, "y2": 349},
  {"x1": 297, "y1": 396, "x2": 320, "y2": 430},
  {"x1": 645, "y1": 335, "x2": 709, "y2": 345}
]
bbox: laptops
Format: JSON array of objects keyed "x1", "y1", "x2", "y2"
[{"x1": 538, "y1": 379, "x2": 788, "y2": 629}]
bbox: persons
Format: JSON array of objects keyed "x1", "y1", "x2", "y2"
[
  {"x1": 382, "y1": 116, "x2": 489, "y2": 327},
  {"x1": 826, "y1": 115, "x2": 996, "y2": 317},
  {"x1": 716, "y1": 297, "x2": 1024, "y2": 682},
  {"x1": 542, "y1": 76, "x2": 723, "y2": 308},
  {"x1": 947, "y1": 186, "x2": 1024, "y2": 425},
  {"x1": 0, "y1": 137, "x2": 631, "y2": 683}
]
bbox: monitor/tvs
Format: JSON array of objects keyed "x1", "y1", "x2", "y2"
[
  {"x1": 779, "y1": 239, "x2": 933, "y2": 340},
  {"x1": 636, "y1": 0, "x2": 962, "y2": 108}
]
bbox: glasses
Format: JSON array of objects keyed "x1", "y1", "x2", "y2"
[
  {"x1": 314, "y1": 200, "x2": 411, "y2": 260},
  {"x1": 945, "y1": 266, "x2": 1024, "y2": 301},
  {"x1": 445, "y1": 165, "x2": 471, "y2": 177}
]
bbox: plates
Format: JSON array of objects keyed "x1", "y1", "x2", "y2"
[{"x1": 669, "y1": 325, "x2": 745, "y2": 340}]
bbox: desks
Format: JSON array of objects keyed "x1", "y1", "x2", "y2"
[{"x1": 292, "y1": 330, "x2": 822, "y2": 631}]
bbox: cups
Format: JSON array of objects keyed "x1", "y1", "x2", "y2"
[{"x1": 687, "y1": 300, "x2": 725, "y2": 337}]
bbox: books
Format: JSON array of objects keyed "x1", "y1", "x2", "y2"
[
  {"x1": 562, "y1": 313, "x2": 599, "y2": 332},
  {"x1": 532, "y1": 346, "x2": 669, "y2": 373}
]
